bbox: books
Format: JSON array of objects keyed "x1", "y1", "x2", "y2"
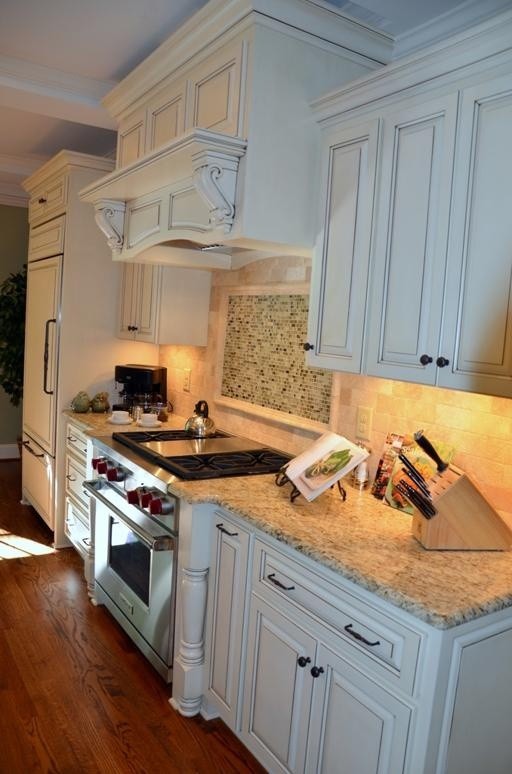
[
  {"x1": 372, "y1": 432, "x2": 453, "y2": 516},
  {"x1": 281, "y1": 430, "x2": 368, "y2": 504}
]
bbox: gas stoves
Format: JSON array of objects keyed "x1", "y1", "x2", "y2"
[{"x1": 94, "y1": 429, "x2": 301, "y2": 533}]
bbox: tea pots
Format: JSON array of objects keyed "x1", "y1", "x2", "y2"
[{"x1": 185, "y1": 400, "x2": 216, "y2": 437}]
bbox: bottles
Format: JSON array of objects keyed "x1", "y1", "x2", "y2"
[
  {"x1": 352, "y1": 434, "x2": 394, "y2": 500},
  {"x1": 71, "y1": 390, "x2": 110, "y2": 413}
]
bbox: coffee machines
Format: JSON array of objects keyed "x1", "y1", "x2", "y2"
[{"x1": 108, "y1": 363, "x2": 169, "y2": 427}]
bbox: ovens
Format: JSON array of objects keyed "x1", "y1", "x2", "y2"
[{"x1": 89, "y1": 481, "x2": 178, "y2": 685}]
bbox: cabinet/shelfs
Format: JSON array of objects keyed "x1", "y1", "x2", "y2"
[
  {"x1": 202, "y1": 507, "x2": 255, "y2": 734},
  {"x1": 20, "y1": 148, "x2": 116, "y2": 549},
  {"x1": 304, "y1": 64, "x2": 381, "y2": 374},
  {"x1": 238, "y1": 527, "x2": 512, "y2": 774},
  {"x1": 360, "y1": 0, "x2": 511, "y2": 400},
  {"x1": 117, "y1": 264, "x2": 211, "y2": 347},
  {"x1": 64, "y1": 422, "x2": 92, "y2": 558}
]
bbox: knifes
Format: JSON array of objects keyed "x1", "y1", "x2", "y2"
[{"x1": 393, "y1": 429, "x2": 447, "y2": 520}]
